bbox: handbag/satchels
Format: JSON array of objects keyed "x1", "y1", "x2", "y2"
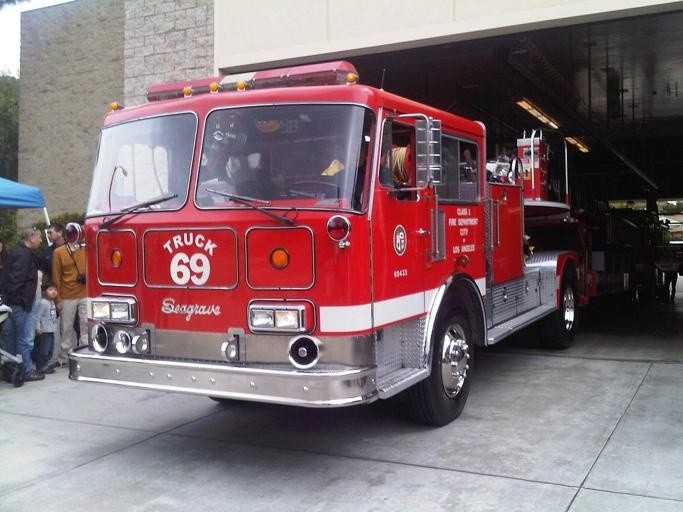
[{"x1": 77, "y1": 274, "x2": 85, "y2": 284}]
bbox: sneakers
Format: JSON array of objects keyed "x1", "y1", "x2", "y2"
[{"x1": 25, "y1": 367, "x2": 55, "y2": 381}]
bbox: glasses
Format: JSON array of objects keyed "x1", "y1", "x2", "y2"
[{"x1": 32, "y1": 226, "x2": 37, "y2": 234}]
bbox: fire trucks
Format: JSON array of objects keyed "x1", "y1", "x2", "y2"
[{"x1": 65, "y1": 60, "x2": 683, "y2": 426}]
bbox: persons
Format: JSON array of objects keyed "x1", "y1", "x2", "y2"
[{"x1": 0, "y1": 222, "x2": 85, "y2": 381}]
